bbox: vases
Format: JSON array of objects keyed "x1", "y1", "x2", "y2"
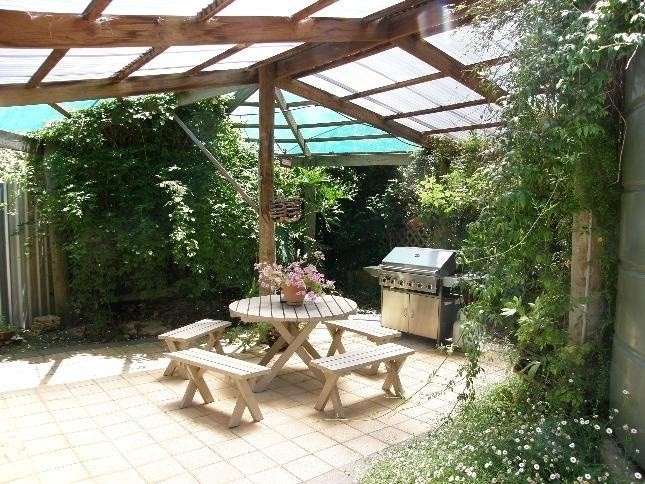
[{"x1": 284, "y1": 289, "x2": 304, "y2": 306}]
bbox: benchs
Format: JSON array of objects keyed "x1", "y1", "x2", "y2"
[
  {"x1": 309, "y1": 342, "x2": 416, "y2": 419},
  {"x1": 157, "y1": 318, "x2": 232, "y2": 380},
  {"x1": 163, "y1": 347, "x2": 272, "y2": 428},
  {"x1": 321, "y1": 321, "x2": 402, "y2": 375}
]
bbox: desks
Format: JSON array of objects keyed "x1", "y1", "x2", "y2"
[{"x1": 227, "y1": 294, "x2": 358, "y2": 393}]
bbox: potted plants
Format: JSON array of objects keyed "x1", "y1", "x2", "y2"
[
  {"x1": 0, "y1": 309, "x2": 21, "y2": 343},
  {"x1": 256, "y1": 322, "x2": 305, "y2": 352}
]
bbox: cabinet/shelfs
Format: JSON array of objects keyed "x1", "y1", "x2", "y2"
[{"x1": 381, "y1": 290, "x2": 440, "y2": 339}]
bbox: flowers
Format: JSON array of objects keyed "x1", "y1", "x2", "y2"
[{"x1": 253, "y1": 249, "x2": 336, "y2": 304}]
